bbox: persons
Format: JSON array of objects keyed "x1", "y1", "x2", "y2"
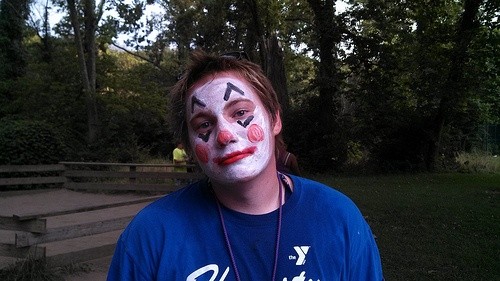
[
  {"x1": 173, "y1": 140, "x2": 193, "y2": 188},
  {"x1": 105, "y1": 56, "x2": 384, "y2": 281},
  {"x1": 277, "y1": 136, "x2": 302, "y2": 177}
]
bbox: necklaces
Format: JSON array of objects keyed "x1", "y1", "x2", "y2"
[{"x1": 214, "y1": 173, "x2": 282, "y2": 281}]
